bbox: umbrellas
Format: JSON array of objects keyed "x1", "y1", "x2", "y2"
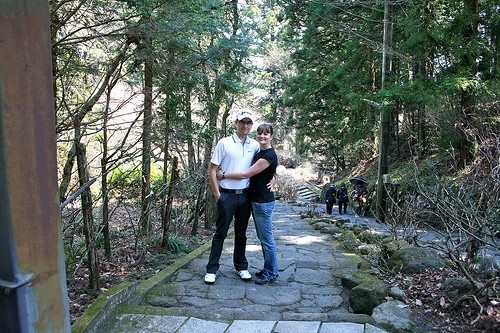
[{"x1": 350, "y1": 176, "x2": 368, "y2": 187}]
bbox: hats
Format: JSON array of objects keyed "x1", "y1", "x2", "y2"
[
  {"x1": 329, "y1": 184, "x2": 335, "y2": 187},
  {"x1": 236, "y1": 109, "x2": 254, "y2": 123},
  {"x1": 341, "y1": 183, "x2": 346, "y2": 187}
]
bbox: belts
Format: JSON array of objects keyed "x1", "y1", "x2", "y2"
[{"x1": 219, "y1": 188, "x2": 247, "y2": 195}]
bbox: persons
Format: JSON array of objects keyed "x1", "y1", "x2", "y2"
[
  {"x1": 337, "y1": 183, "x2": 348, "y2": 215},
  {"x1": 203, "y1": 110, "x2": 276, "y2": 284},
  {"x1": 325, "y1": 184, "x2": 337, "y2": 215},
  {"x1": 216, "y1": 124, "x2": 278, "y2": 285},
  {"x1": 351, "y1": 186, "x2": 368, "y2": 217}
]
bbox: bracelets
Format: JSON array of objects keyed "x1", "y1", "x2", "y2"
[
  {"x1": 223, "y1": 172, "x2": 225, "y2": 178},
  {"x1": 273, "y1": 175, "x2": 276, "y2": 178}
]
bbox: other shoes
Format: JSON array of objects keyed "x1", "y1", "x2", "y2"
[{"x1": 254, "y1": 268, "x2": 277, "y2": 285}]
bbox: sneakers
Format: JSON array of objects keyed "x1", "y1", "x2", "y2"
[
  {"x1": 204, "y1": 273, "x2": 216, "y2": 283},
  {"x1": 235, "y1": 269, "x2": 251, "y2": 279}
]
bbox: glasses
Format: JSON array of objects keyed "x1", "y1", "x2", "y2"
[{"x1": 238, "y1": 120, "x2": 252, "y2": 124}]
